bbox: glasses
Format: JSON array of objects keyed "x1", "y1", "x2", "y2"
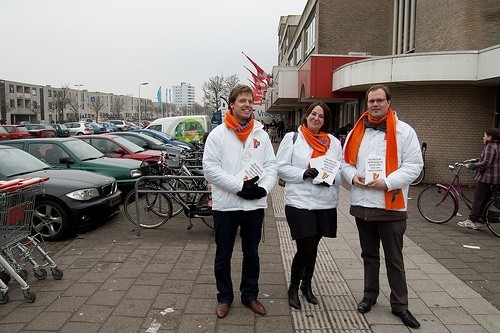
[{"x1": 368, "y1": 99, "x2": 387, "y2": 103}]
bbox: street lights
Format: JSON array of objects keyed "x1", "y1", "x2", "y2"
[
  {"x1": 139, "y1": 82, "x2": 148, "y2": 130},
  {"x1": 75, "y1": 85, "x2": 84, "y2": 121}
]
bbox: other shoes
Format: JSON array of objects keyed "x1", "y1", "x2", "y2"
[{"x1": 457, "y1": 219, "x2": 478, "y2": 230}]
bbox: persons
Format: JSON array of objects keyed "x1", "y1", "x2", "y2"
[
  {"x1": 278, "y1": 118, "x2": 285, "y2": 139},
  {"x1": 340, "y1": 85, "x2": 422, "y2": 329},
  {"x1": 328, "y1": 123, "x2": 352, "y2": 148},
  {"x1": 275, "y1": 101, "x2": 343, "y2": 309},
  {"x1": 202, "y1": 85, "x2": 278, "y2": 318},
  {"x1": 458, "y1": 128, "x2": 500, "y2": 232}
]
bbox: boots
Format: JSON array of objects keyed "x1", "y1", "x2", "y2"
[
  {"x1": 287, "y1": 282, "x2": 301, "y2": 309},
  {"x1": 300, "y1": 279, "x2": 318, "y2": 304}
]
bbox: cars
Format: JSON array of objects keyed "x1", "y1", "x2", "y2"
[
  {"x1": 12, "y1": 117, "x2": 153, "y2": 138},
  {"x1": 127, "y1": 129, "x2": 197, "y2": 152},
  {"x1": 0, "y1": 137, "x2": 152, "y2": 204},
  {"x1": 0, "y1": 144, "x2": 124, "y2": 242},
  {"x1": 39, "y1": 133, "x2": 167, "y2": 185},
  {"x1": 0, "y1": 125, "x2": 32, "y2": 141},
  {"x1": 100, "y1": 131, "x2": 195, "y2": 169}
]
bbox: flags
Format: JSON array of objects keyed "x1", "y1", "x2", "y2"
[{"x1": 242, "y1": 53, "x2": 270, "y2": 106}]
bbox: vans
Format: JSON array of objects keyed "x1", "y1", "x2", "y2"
[{"x1": 140, "y1": 114, "x2": 212, "y2": 151}]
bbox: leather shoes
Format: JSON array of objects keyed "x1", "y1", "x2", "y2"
[
  {"x1": 357, "y1": 297, "x2": 377, "y2": 313},
  {"x1": 215, "y1": 303, "x2": 230, "y2": 318},
  {"x1": 248, "y1": 298, "x2": 266, "y2": 315},
  {"x1": 392, "y1": 309, "x2": 421, "y2": 329}
]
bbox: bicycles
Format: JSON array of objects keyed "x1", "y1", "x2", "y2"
[
  {"x1": 416, "y1": 160, "x2": 500, "y2": 238},
  {"x1": 410, "y1": 142, "x2": 427, "y2": 187},
  {"x1": 123, "y1": 144, "x2": 215, "y2": 230}
]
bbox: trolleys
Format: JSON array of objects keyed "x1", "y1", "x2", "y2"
[{"x1": 0, "y1": 175, "x2": 64, "y2": 305}]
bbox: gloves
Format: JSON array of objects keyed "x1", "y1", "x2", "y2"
[
  {"x1": 303, "y1": 168, "x2": 319, "y2": 180},
  {"x1": 318, "y1": 179, "x2": 336, "y2": 188},
  {"x1": 237, "y1": 176, "x2": 267, "y2": 200}
]
